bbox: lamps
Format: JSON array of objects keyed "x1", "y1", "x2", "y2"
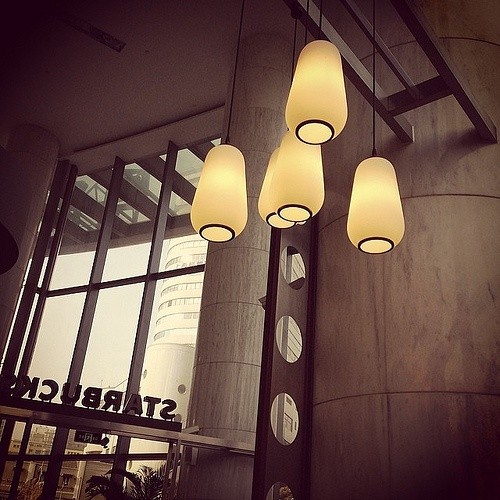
[
  {"x1": 284, "y1": 0, "x2": 350, "y2": 146},
  {"x1": 345, "y1": 0, "x2": 405, "y2": 255},
  {"x1": 258, "y1": 146, "x2": 297, "y2": 229},
  {"x1": 190, "y1": 0, "x2": 249, "y2": 243},
  {"x1": 274, "y1": 0, "x2": 326, "y2": 223}
]
